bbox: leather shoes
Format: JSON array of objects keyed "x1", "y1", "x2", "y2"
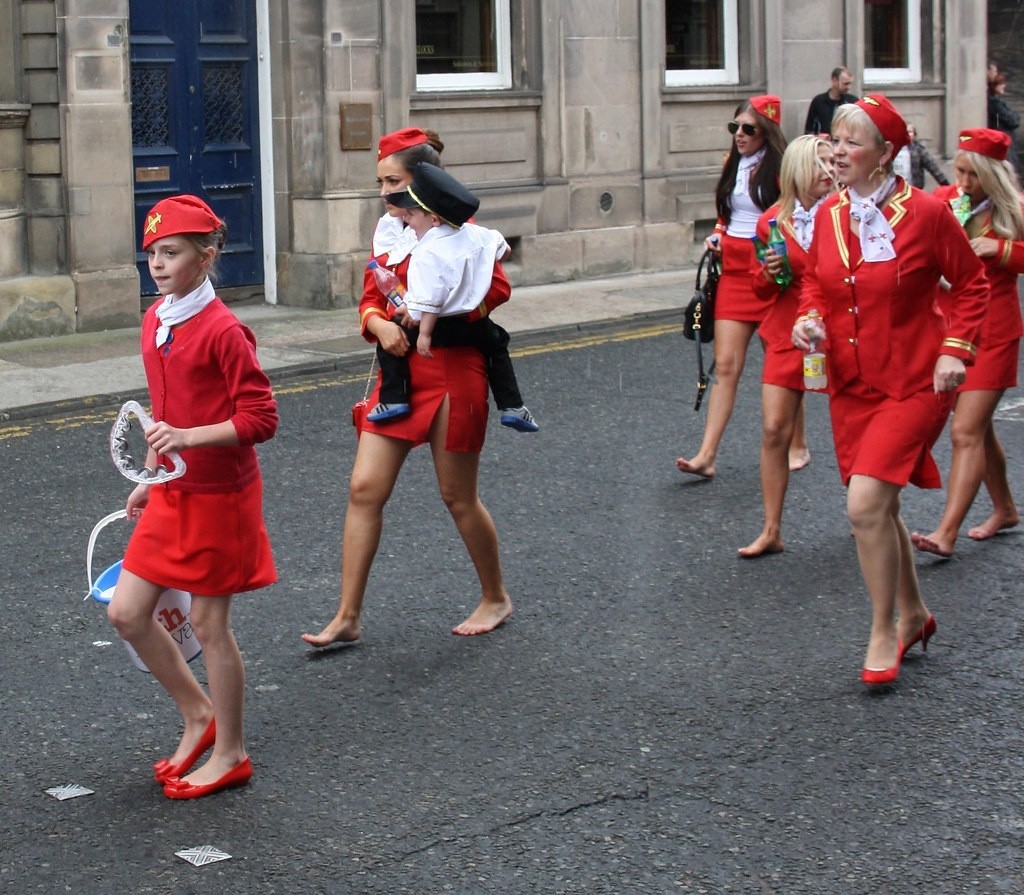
[
  {"x1": 153, "y1": 711, "x2": 215, "y2": 786},
  {"x1": 163, "y1": 755, "x2": 252, "y2": 800}
]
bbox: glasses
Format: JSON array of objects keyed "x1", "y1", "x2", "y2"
[{"x1": 727, "y1": 120, "x2": 761, "y2": 136}]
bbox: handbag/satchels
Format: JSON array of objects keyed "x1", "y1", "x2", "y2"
[
  {"x1": 352, "y1": 398, "x2": 372, "y2": 439},
  {"x1": 683, "y1": 246, "x2": 720, "y2": 343}
]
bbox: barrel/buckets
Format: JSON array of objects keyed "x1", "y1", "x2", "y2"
[{"x1": 84, "y1": 507, "x2": 203, "y2": 674}]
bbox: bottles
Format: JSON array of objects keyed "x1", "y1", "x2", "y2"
[
  {"x1": 368, "y1": 261, "x2": 407, "y2": 307},
  {"x1": 801, "y1": 309, "x2": 827, "y2": 390},
  {"x1": 710, "y1": 238, "x2": 722, "y2": 276},
  {"x1": 768, "y1": 219, "x2": 793, "y2": 285},
  {"x1": 751, "y1": 236, "x2": 768, "y2": 265},
  {"x1": 950, "y1": 193, "x2": 972, "y2": 227}
]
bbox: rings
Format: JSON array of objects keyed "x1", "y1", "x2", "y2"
[
  {"x1": 795, "y1": 342, "x2": 798, "y2": 346},
  {"x1": 953, "y1": 380, "x2": 958, "y2": 389}
]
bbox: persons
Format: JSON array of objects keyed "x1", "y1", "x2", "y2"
[
  {"x1": 301, "y1": 127, "x2": 512, "y2": 647},
  {"x1": 911, "y1": 127, "x2": 1024, "y2": 557},
  {"x1": 789, "y1": 95, "x2": 992, "y2": 683},
  {"x1": 677, "y1": 95, "x2": 810, "y2": 478},
  {"x1": 739, "y1": 134, "x2": 854, "y2": 555},
  {"x1": 892, "y1": 125, "x2": 950, "y2": 191},
  {"x1": 986, "y1": 63, "x2": 1020, "y2": 165},
  {"x1": 107, "y1": 195, "x2": 280, "y2": 798},
  {"x1": 367, "y1": 162, "x2": 540, "y2": 432},
  {"x1": 804, "y1": 66, "x2": 860, "y2": 137}
]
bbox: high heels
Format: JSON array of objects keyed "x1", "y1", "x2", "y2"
[
  {"x1": 902, "y1": 613, "x2": 936, "y2": 659},
  {"x1": 861, "y1": 635, "x2": 904, "y2": 684}
]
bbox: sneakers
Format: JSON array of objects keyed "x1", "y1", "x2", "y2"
[
  {"x1": 366, "y1": 399, "x2": 409, "y2": 421},
  {"x1": 500, "y1": 405, "x2": 539, "y2": 432}
]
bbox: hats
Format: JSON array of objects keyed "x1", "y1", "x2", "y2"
[
  {"x1": 385, "y1": 162, "x2": 480, "y2": 227},
  {"x1": 378, "y1": 127, "x2": 428, "y2": 160},
  {"x1": 958, "y1": 128, "x2": 1011, "y2": 160},
  {"x1": 143, "y1": 194, "x2": 223, "y2": 251},
  {"x1": 854, "y1": 92, "x2": 912, "y2": 161},
  {"x1": 749, "y1": 94, "x2": 781, "y2": 126}
]
